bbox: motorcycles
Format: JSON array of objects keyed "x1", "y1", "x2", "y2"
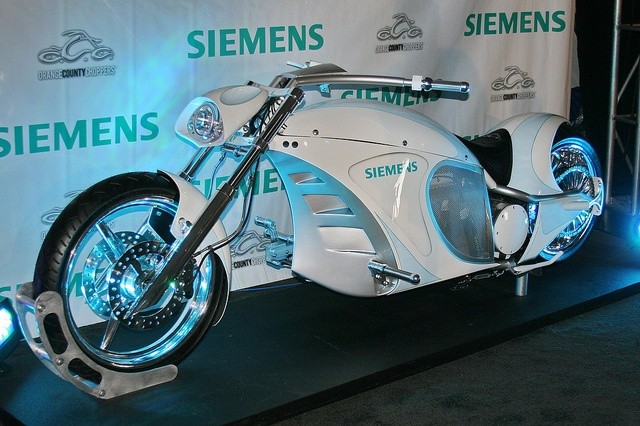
[{"x1": 1, "y1": 58, "x2": 610, "y2": 399}]
